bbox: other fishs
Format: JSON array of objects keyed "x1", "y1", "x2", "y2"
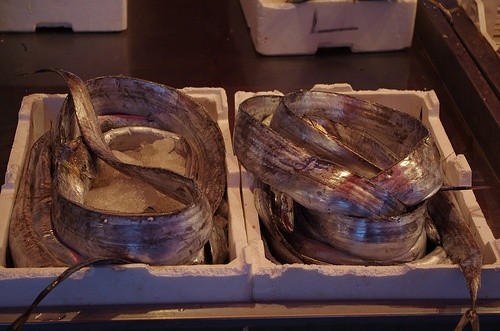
[
  {"x1": 8, "y1": 65, "x2": 231, "y2": 331},
  {"x1": 232, "y1": 88, "x2": 484, "y2": 330}
]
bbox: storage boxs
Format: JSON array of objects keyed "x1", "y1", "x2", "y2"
[
  {"x1": 0, "y1": 0, "x2": 128, "y2": 32},
  {"x1": 240, "y1": 0, "x2": 417, "y2": 55},
  {"x1": 0, "y1": 87, "x2": 253, "y2": 307},
  {"x1": 234, "y1": 82, "x2": 500, "y2": 301}
]
